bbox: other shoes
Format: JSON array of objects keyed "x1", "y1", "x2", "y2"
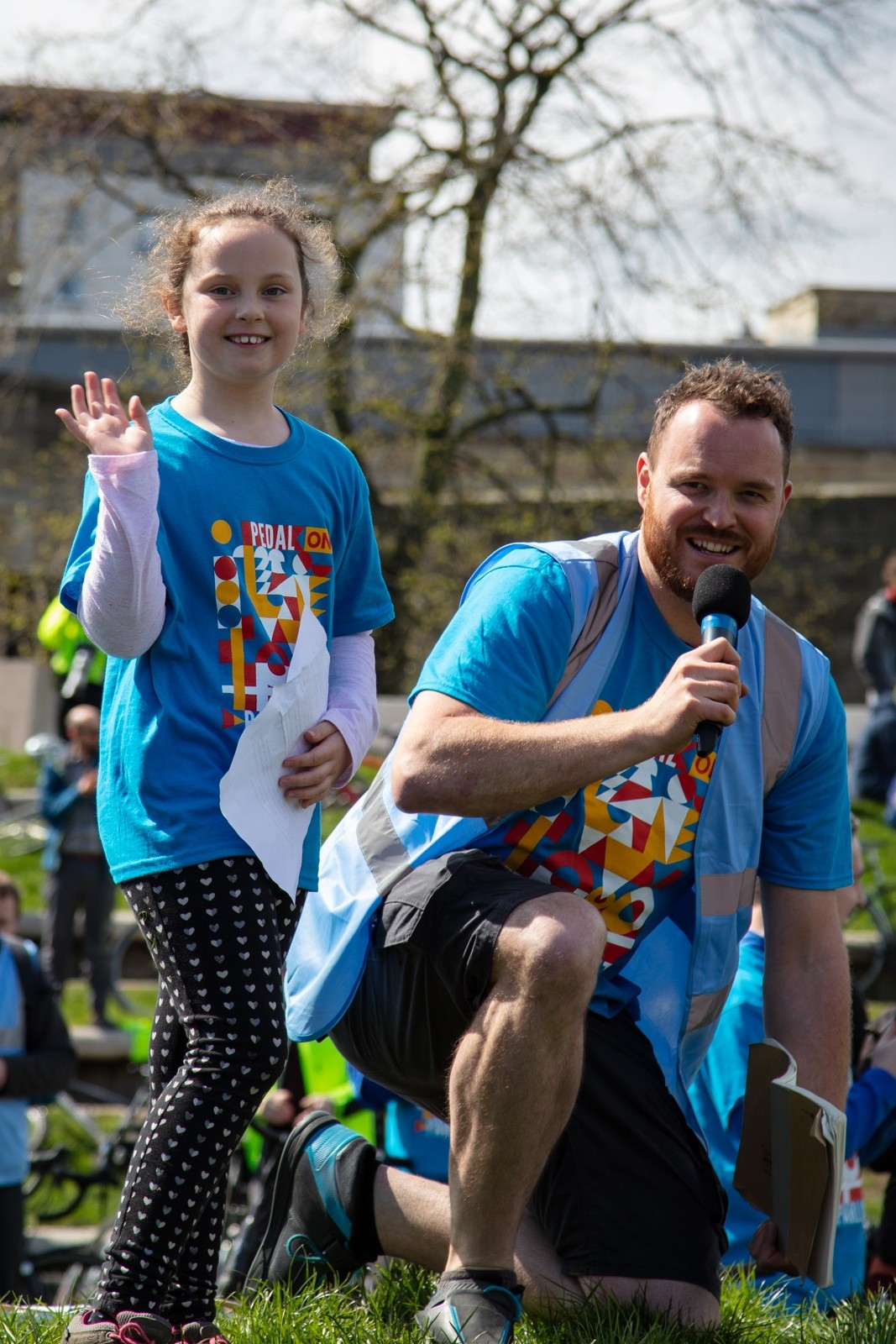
[{"x1": 62, "y1": 1303, "x2": 228, "y2": 1344}]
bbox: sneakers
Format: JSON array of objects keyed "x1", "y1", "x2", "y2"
[
  {"x1": 241, "y1": 1108, "x2": 377, "y2": 1303},
  {"x1": 413, "y1": 1280, "x2": 524, "y2": 1344}
]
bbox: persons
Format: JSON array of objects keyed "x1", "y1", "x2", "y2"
[
  {"x1": 0, "y1": 873, "x2": 65, "y2": 1306},
  {"x1": 36, "y1": 704, "x2": 118, "y2": 1030},
  {"x1": 39, "y1": 593, "x2": 107, "y2": 739},
  {"x1": 219, "y1": 352, "x2": 896, "y2": 1344},
  {"x1": 52, "y1": 195, "x2": 393, "y2": 1344},
  {"x1": 258, "y1": 1028, "x2": 454, "y2": 1185}
]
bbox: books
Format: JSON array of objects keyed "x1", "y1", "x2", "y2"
[{"x1": 730, "y1": 1039, "x2": 847, "y2": 1296}]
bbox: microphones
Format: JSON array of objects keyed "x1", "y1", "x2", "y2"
[{"x1": 692, "y1": 564, "x2": 753, "y2": 759}]
